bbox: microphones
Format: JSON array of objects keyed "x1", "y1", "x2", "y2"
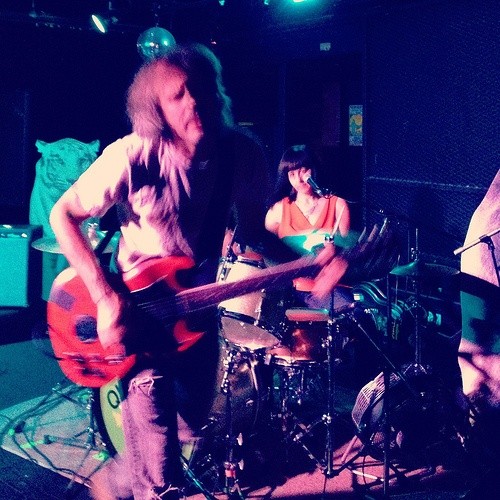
[{"x1": 303, "y1": 174, "x2": 323, "y2": 196}]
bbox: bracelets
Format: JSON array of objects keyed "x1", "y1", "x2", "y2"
[{"x1": 95, "y1": 289, "x2": 113, "y2": 305}]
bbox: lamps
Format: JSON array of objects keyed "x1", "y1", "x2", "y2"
[
  {"x1": 91, "y1": 2, "x2": 119, "y2": 34},
  {"x1": 137, "y1": 22, "x2": 177, "y2": 62}
]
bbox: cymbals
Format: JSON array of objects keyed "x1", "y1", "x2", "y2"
[
  {"x1": 388, "y1": 262, "x2": 461, "y2": 279},
  {"x1": 32, "y1": 230, "x2": 118, "y2": 256}
]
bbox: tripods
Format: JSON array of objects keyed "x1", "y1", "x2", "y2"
[
  {"x1": 291, "y1": 322, "x2": 356, "y2": 480},
  {"x1": 43, "y1": 390, "x2": 111, "y2": 497}
]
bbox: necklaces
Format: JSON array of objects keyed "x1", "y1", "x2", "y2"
[{"x1": 305, "y1": 198, "x2": 319, "y2": 221}]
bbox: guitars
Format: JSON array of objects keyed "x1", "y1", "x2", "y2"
[{"x1": 47, "y1": 218, "x2": 394, "y2": 388}]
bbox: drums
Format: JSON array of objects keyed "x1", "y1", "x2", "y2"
[
  {"x1": 303, "y1": 288, "x2": 354, "y2": 313},
  {"x1": 264, "y1": 307, "x2": 330, "y2": 366},
  {"x1": 88, "y1": 334, "x2": 275, "y2": 481},
  {"x1": 215, "y1": 253, "x2": 307, "y2": 348}
]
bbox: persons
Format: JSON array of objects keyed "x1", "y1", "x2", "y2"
[
  {"x1": 457, "y1": 172, "x2": 500, "y2": 427},
  {"x1": 264, "y1": 145, "x2": 349, "y2": 242},
  {"x1": 48, "y1": 44, "x2": 349, "y2": 499}
]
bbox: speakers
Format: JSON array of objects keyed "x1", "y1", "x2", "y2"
[{"x1": 0, "y1": 225, "x2": 43, "y2": 308}]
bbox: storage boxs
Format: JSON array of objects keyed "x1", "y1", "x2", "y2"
[{"x1": 0, "y1": 224, "x2": 43, "y2": 309}]
